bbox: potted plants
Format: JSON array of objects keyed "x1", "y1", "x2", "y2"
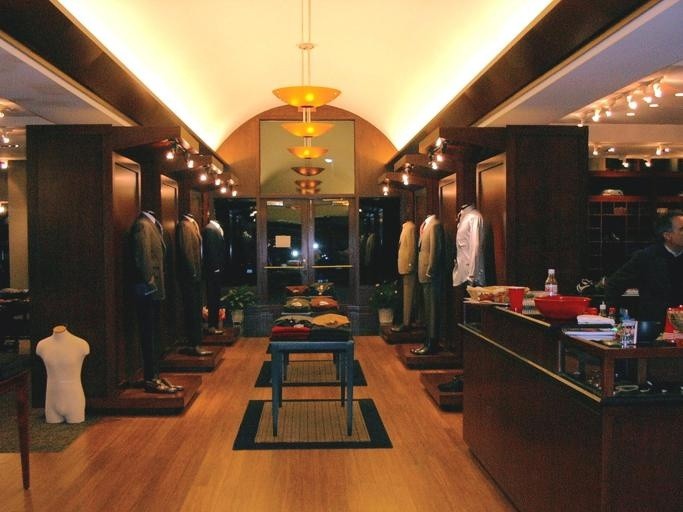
[{"x1": 220, "y1": 284, "x2": 256, "y2": 325}]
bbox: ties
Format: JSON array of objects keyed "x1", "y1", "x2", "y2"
[
  {"x1": 193, "y1": 221, "x2": 199, "y2": 236},
  {"x1": 454, "y1": 212, "x2": 461, "y2": 261},
  {"x1": 420, "y1": 221, "x2": 426, "y2": 248},
  {"x1": 155, "y1": 218, "x2": 163, "y2": 241}
]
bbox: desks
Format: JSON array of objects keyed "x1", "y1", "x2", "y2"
[{"x1": 263, "y1": 289, "x2": 356, "y2": 440}]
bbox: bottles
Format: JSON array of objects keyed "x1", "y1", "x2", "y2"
[
  {"x1": 598, "y1": 300, "x2": 630, "y2": 324},
  {"x1": 545, "y1": 268, "x2": 558, "y2": 295}
]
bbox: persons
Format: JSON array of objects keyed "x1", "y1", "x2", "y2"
[
  {"x1": 390, "y1": 220, "x2": 419, "y2": 333},
  {"x1": 128, "y1": 209, "x2": 184, "y2": 394},
  {"x1": 437, "y1": 201, "x2": 486, "y2": 392},
  {"x1": 410, "y1": 214, "x2": 445, "y2": 355},
  {"x1": 174, "y1": 213, "x2": 214, "y2": 356},
  {"x1": 201, "y1": 219, "x2": 230, "y2": 335},
  {"x1": 582, "y1": 208, "x2": 683, "y2": 333},
  {"x1": 35, "y1": 325, "x2": 90, "y2": 424}
]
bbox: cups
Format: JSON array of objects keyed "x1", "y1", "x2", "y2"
[
  {"x1": 621, "y1": 320, "x2": 638, "y2": 345},
  {"x1": 507, "y1": 288, "x2": 525, "y2": 314}
]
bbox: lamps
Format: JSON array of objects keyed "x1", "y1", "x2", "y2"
[
  {"x1": 164, "y1": 139, "x2": 239, "y2": 199},
  {"x1": 571, "y1": 72, "x2": 663, "y2": 128},
  {"x1": 0, "y1": 99, "x2": 24, "y2": 215},
  {"x1": 381, "y1": 139, "x2": 455, "y2": 199},
  {"x1": 592, "y1": 144, "x2": 672, "y2": 169},
  {"x1": 270, "y1": 0, "x2": 341, "y2": 193}
]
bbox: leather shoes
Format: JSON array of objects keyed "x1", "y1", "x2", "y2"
[
  {"x1": 391, "y1": 323, "x2": 414, "y2": 332},
  {"x1": 183, "y1": 346, "x2": 215, "y2": 357},
  {"x1": 438, "y1": 375, "x2": 463, "y2": 392},
  {"x1": 410, "y1": 346, "x2": 443, "y2": 355},
  {"x1": 145, "y1": 378, "x2": 185, "y2": 395},
  {"x1": 204, "y1": 326, "x2": 225, "y2": 336}
]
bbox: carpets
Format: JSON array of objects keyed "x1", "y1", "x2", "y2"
[
  {"x1": 0, "y1": 390, "x2": 104, "y2": 455},
  {"x1": 253, "y1": 359, "x2": 367, "y2": 387},
  {"x1": 231, "y1": 398, "x2": 393, "y2": 450}
]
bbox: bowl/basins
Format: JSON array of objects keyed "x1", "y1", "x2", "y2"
[
  {"x1": 535, "y1": 295, "x2": 593, "y2": 319},
  {"x1": 637, "y1": 322, "x2": 663, "y2": 342},
  {"x1": 667, "y1": 311, "x2": 683, "y2": 332}
]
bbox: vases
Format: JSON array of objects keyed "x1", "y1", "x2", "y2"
[{"x1": 376, "y1": 306, "x2": 394, "y2": 326}]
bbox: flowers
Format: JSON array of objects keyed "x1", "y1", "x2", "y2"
[{"x1": 367, "y1": 278, "x2": 401, "y2": 310}]
